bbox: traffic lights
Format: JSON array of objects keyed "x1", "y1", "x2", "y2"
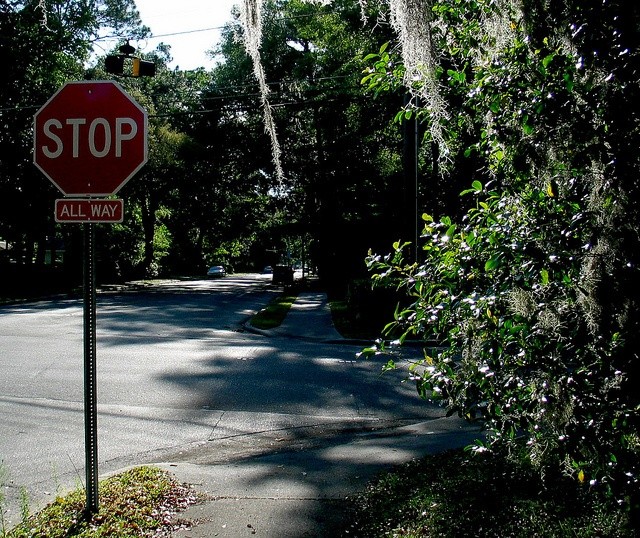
[
  {"x1": 105, "y1": 55, "x2": 124, "y2": 74},
  {"x1": 133, "y1": 59, "x2": 155, "y2": 76}
]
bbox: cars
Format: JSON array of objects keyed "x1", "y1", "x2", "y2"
[
  {"x1": 207, "y1": 266, "x2": 225, "y2": 277},
  {"x1": 264, "y1": 266, "x2": 274, "y2": 274}
]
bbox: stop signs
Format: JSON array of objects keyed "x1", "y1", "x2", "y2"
[{"x1": 34, "y1": 80, "x2": 147, "y2": 197}]
